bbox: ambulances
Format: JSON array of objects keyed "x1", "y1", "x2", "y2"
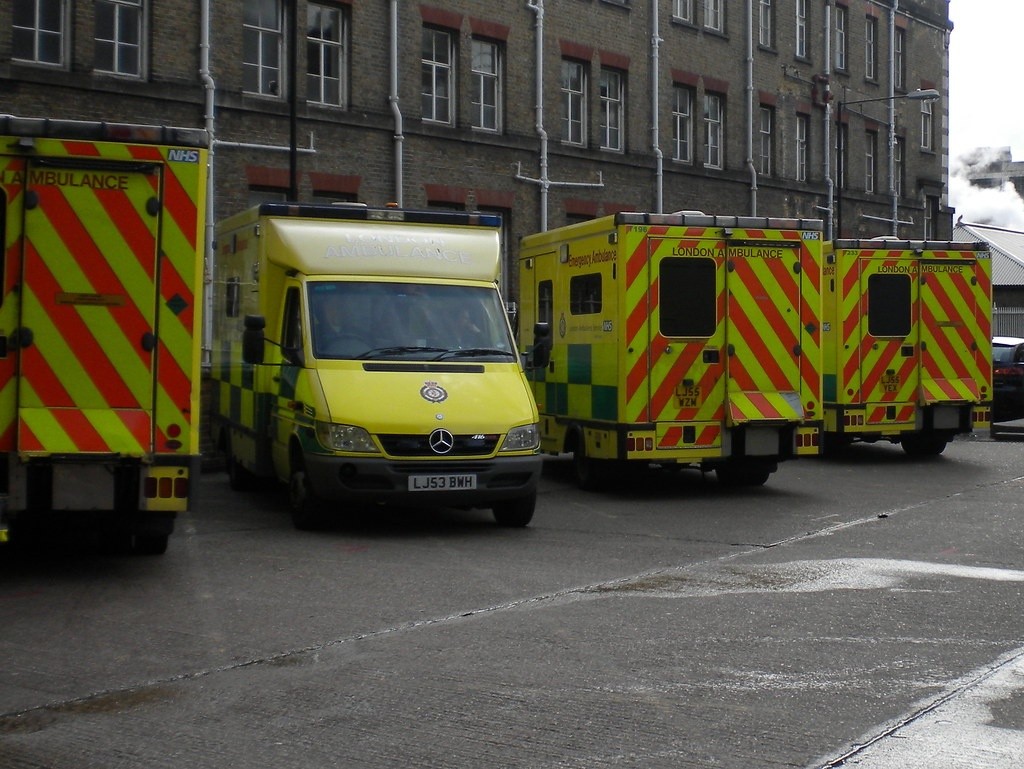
[
  {"x1": 514, "y1": 209, "x2": 826, "y2": 495},
  {"x1": 209, "y1": 199, "x2": 552, "y2": 533},
  {"x1": 822, "y1": 236, "x2": 997, "y2": 461},
  {"x1": 0, "y1": 109, "x2": 212, "y2": 556}
]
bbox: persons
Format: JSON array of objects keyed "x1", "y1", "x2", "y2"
[
  {"x1": 312, "y1": 293, "x2": 371, "y2": 354},
  {"x1": 437, "y1": 311, "x2": 492, "y2": 349}
]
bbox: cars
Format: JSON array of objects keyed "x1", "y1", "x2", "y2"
[{"x1": 992, "y1": 336, "x2": 1024, "y2": 425}]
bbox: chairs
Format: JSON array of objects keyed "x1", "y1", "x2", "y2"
[{"x1": 1000, "y1": 352, "x2": 1009, "y2": 362}]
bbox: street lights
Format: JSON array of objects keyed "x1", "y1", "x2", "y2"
[{"x1": 836, "y1": 88, "x2": 941, "y2": 238}]
